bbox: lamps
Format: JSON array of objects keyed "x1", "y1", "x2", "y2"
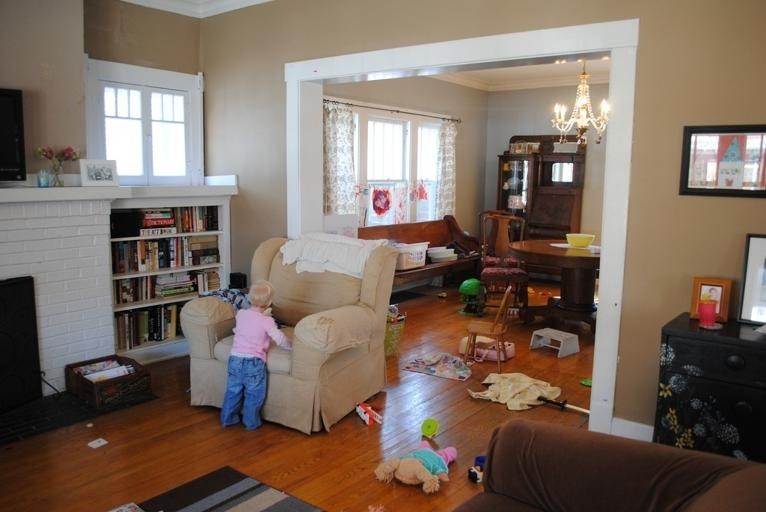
[{"x1": 549, "y1": 60, "x2": 610, "y2": 147}]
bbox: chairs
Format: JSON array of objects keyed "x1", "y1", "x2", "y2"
[
  {"x1": 179, "y1": 236, "x2": 398, "y2": 435},
  {"x1": 462, "y1": 286, "x2": 516, "y2": 373},
  {"x1": 479, "y1": 210, "x2": 530, "y2": 318}
]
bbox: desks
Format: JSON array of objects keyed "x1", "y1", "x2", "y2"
[{"x1": 508, "y1": 238, "x2": 601, "y2": 334}]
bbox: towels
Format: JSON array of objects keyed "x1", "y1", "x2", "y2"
[{"x1": 400, "y1": 351, "x2": 472, "y2": 381}]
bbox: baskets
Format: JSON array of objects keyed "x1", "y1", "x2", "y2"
[{"x1": 396, "y1": 241, "x2": 430, "y2": 271}]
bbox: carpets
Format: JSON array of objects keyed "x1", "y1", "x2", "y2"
[{"x1": 101, "y1": 466, "x2": 327, "y2": 512}]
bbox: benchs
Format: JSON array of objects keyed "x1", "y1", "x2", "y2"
[{"x1": 358, "y1": 214, "x2": 485, "y2": 292}]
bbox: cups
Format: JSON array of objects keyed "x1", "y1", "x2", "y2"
[
  {"x1": 37, "y1": 168, "x2": 49, "y2": 188},
  {"x1": 701, "y1": 298, "x2": 717, "y2": 327},
  {"x1": 503, "y1": 163, "x2": 509, "y2": 171}
]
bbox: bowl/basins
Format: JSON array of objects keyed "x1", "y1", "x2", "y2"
[{"x1": 565, "y1": 234, "x2": 594, "y2": 249}]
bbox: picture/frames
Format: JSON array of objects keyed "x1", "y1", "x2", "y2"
[
  {"x1": 688, "y1": 233, "x2": 766, "y2": 325},
  {"x1": 78, "y1": 158, "x2": 118, "y2": 186},
  {"x1": 678, "y1": 123, "x2": 766, "y2": 199}
]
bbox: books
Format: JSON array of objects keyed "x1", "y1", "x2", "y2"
[
  {"x1": 108, "y1": 205, "x2": 223, "y2": 352},
  {"x1": 70, "y1": 358, "x2": 136, "y2": 384}
]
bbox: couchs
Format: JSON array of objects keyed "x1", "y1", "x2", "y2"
[{"x1": 447, "y1": 418, "x2": 764, "y2": 512}]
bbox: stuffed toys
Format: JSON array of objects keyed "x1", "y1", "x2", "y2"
[
  {"x1": 466, "y1": 452, "x2": 486, "y2": 484},
  {"x1": 373, "y1": 439, "x2": 459, "y2": 494}
]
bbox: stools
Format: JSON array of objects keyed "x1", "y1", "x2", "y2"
[{"x1": 530, "y1": 328, "x2": 580, "y2": 358}]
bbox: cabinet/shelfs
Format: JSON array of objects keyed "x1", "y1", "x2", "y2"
[
  {"x1": 109, "y1": 195, "x2": 230, "y2": 366},
  {"x1": 497, "y1": 152, "x2": 585, "y2": 249},
  {"x1": 652, "y1": 335, "x2": 765, "y2": 463}
]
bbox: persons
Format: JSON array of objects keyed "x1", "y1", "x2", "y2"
[
  {"x1": 706, "y1": 286, "x2": 718, "y2": 300},
  {"x1": 220, "y1": 280, "x2": 293, "y2": 432}
]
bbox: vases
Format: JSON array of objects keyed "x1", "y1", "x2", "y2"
[{"x1": 48, "y1": 170, "x2": 64, "y2": 185}]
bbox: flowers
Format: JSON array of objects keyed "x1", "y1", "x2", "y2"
[{"x1": 36, "y1": 144, "x2": 82, "y2": 182}]
